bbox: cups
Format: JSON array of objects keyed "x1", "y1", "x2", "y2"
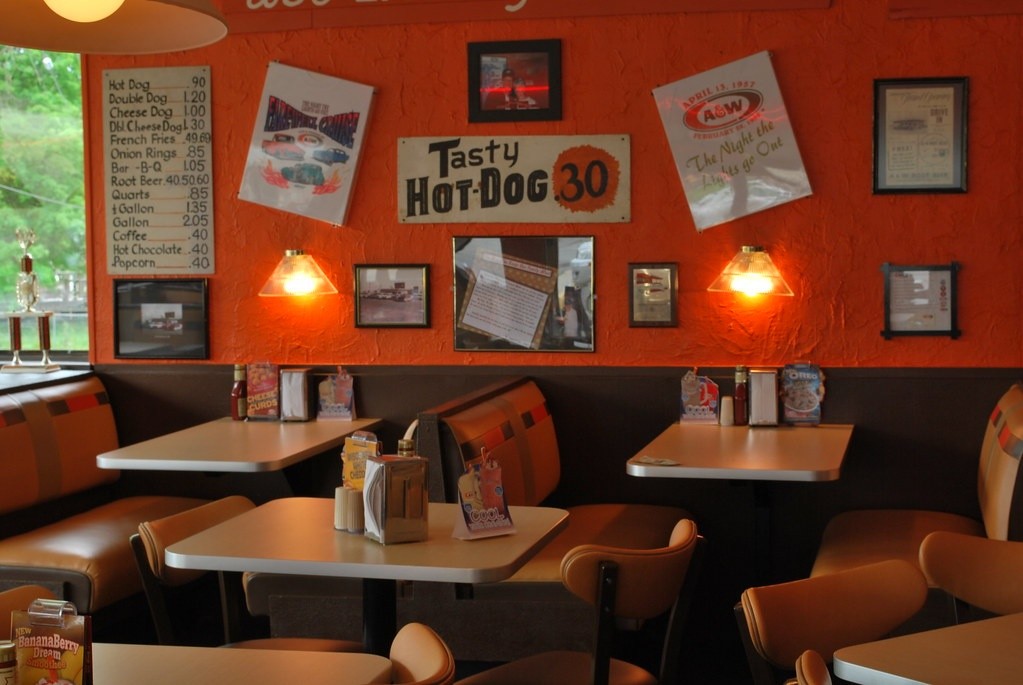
[
  {"x1": 681, "y1": 377, "x2": 718, "y2": 412},
  {"x1": 458, "y1": 458, "x2": 504, "y2": 521},
  {"x1": 318, "y1": 374, "x2": 354, "y2": 408}
]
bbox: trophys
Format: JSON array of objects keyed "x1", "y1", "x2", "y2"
[{"x1": 0, "y1": 226, "x2": 61, "y2": 374}]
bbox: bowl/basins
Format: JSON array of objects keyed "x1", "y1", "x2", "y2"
[{"x1": 783, "y1": 384, "x2": 819, "y2": 413}]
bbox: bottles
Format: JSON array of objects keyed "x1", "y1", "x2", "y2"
[
  {"x1": 230, "y1": 363, "x2": 249, "y2": 421},
  {"x1": 333, "y1": 487, "x2": 353, "y2": 531},
  {"x1": 733, "y1": 364, "x2": 748, "y2": 426},
  {"x1": 720, "y1": 396, "x2": 734, "y2": 425},
  {"x1": 346, "y1": 490, "x2": 366, "y2": 534},
  {"x1": 0, "y1": 642, "x2": 18, "y2": 685},
  {"x1": 397, "y1": 438, "x2": 416, "y2": 458}
]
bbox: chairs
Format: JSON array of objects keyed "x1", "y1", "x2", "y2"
[{"x1": 0, "y1": 489, "x2": 1022, "y2": 685}]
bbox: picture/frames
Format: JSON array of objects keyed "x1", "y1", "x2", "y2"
[
  {"x1": 626, "y1": 261, "x2": 678, "y2": 327},
  {"x1": 452, "y1": 235, "x2": 596, "y2": 353},
  {"x1": 464, "y1": 37, "x2": 563, "y2": 122},
  {"x1": 354, "y1": 263, "x2": 430, "y2": 328},
  {"x1": 113, "y1": 277, "x2": 208, "y2": 361}
]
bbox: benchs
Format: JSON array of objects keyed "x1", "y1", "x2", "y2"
[
  {"x1": 810, "y1": 382, "x2": 1022, "y2": 625},
  {"x1": 414, "y1": 374, "x2": 678, "y2": 600},
  {"x1": 0, "y1": 372, "x2": 208, "y2": 622}
]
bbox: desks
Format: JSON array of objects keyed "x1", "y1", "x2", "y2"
[
  {"x1": 625, "y1": 415, "x2": 856, "y2": 589},
  {"x1": 834, "y1": 611, "x2": 1023, "y2": 685},
  {"x1": 86, "y1": 638, "x2": 393, "y2": 685},
  {"x1": 162, "y1": 495, "x2": 571, "y2": 657},
  {"x1": 94, "y1": 417, "x2": 386, "y2": 498}
]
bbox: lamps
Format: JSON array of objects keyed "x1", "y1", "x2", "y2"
[
  {"x1": 706, "y1": 245, "x2": 795, "y2": 297},
  {"x1": 256, "y1": 248, "x2": 339, "y2": 300},
  {"x1": 1, "y1": 0, "x2": 228, "y2": 56}
]
